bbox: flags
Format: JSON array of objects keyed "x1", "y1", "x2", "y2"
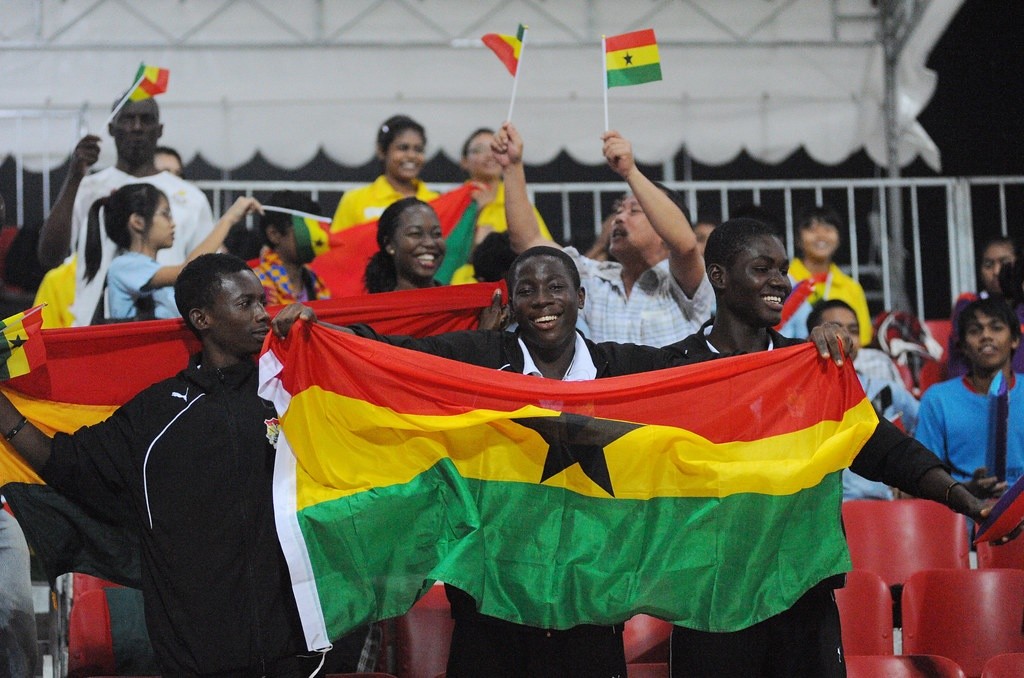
[
  {"x1": 605, "y1": 29, "x2": 663, "y2": 87},
  {"x1": 480, "y1": 24, "x2": 524, "y2": 74},
  {"x1": 246, "y1": 177, "x2": 488, "y2": 306},
  {"x1": 256, "y1": 314, "x2": 882, "y2": 652},
  {"x1": 289, "y1": 212, "x2": 332, "y2": 262},
  {"x1": 0, "y1": 304, "x2": 52, "y2": 400},
  {"x1": 128, "y1": 64, "x2": 169, "y2": 102},
  {"x1": 0, "y1": 280, "x2": 509, "y2": 678}
]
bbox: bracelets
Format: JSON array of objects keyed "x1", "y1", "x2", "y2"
[
  {"x1": 945, "y1": 482, "x2": 971, "y2": 513},
  {"x1": 4, "y1": 417, "x2": 27, "y2": 442}
]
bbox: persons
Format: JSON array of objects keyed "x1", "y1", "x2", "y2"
[{"x1": 0, "y1": 95, "x2": 1024, "y2": 678}]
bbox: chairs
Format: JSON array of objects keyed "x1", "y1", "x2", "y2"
[{"x1": 0, "y1": 225, "x2": 1024, "y2": 678}]
